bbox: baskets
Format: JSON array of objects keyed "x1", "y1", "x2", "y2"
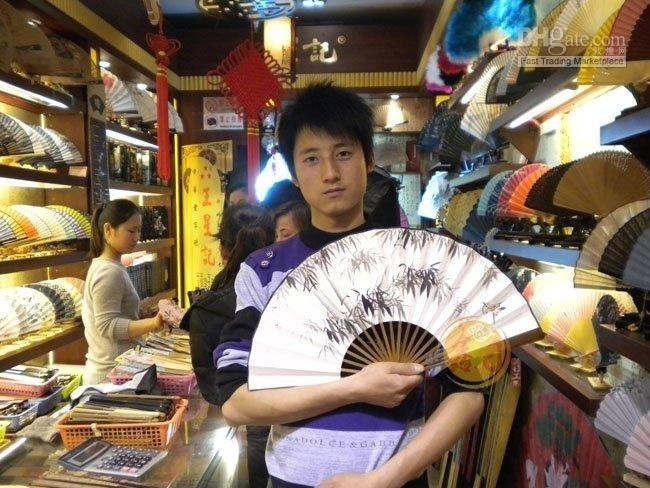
[
  {"x1": 55, "y1": 393, "x2": 188, "y2": 451},
  {"x1": 0, "y1": 365, "x2": 83, "y2": 444},
  {"x1": 107, "y1": 362, "x2": 198, "y2": 398}
]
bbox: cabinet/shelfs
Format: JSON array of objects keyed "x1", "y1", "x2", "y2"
[{"x1": 0, "y1": 0, "x2": 650, "y2": 488}]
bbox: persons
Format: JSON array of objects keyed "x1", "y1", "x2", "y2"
[
  {"x1": 214, "y1": 82, "x2": 487, "y2": 488},
  {"x1": 82, "y1": 199, "x2": 173, "y2": 388},
  {"x1": 226, "y1": 165, "x2": 408, "y2": 241},
  {"x1": 178, "y1": 204, "x2": 276, "y2": 488}
]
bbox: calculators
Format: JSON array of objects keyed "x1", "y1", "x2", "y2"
[{"x1": 57, "y1": 437, "x2": 170, "y2": 481}]
